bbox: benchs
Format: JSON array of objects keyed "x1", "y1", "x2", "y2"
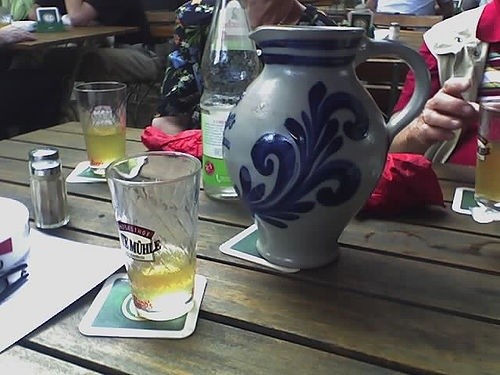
[
  {"x1": 144, "y1": 9, "x2": 177, "y2": 51},
  {"x1": 372, "y1": 12, "x2": 444, "y2": 46}
]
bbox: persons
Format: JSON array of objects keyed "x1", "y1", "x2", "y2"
[
  {"x1": 0, "y1": 0, "x2": 165, "y2": 126},
  {"x1": 161, "y1": 0, "x2": 369, "y2": 130},
  {"x1": 387, "y1": 0, "x2": 500, "y2": 166},
  {"x1": 366, "y1": 0, "x2": 490, "y2": 32}
]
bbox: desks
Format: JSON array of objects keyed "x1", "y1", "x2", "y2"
[
  {"x1": 356, "y1": 44, "x2": 424, "y2": 117},
  {"x1": 0, "y1": 120, "x2": 500, "y2": 375},
  {"x1": 0, "y1": 20, "x2": 139, "y2": 134}
]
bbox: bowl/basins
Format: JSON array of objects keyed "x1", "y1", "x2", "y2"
[
  {"x1": 0, "y1": 197, "x2": 31, "y2": 302},
  {"x1": 11, "y1": 20, "x2": 39, "y2": 32}
]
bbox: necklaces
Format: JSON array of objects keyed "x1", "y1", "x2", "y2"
[{"x1": 277, "y1": 1, "x2": 294, "y2": 25}]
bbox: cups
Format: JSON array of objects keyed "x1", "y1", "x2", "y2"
[
  {"x1": 0, "y1": 6, "x2": 11, "y2": 25},
  {"x1": 105, "y1": 151, "x2": 202, "y2": 322},
  {"x1": 73, "y1": 81, "x2": 128, "y2": 175},
  {"x1": 474, "y1": 96, "x2": 500, "y2": 202}
]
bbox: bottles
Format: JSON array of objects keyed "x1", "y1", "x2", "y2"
[
  {"x1": 200, "y1": 0, "x2": 263, "y2": 201},
  {"x1": 28, "y1": 146, "x2": 70, "y2": 229},
  {"x1": 221, "y1": 26, "x2": 432, "y2": 269}
]
